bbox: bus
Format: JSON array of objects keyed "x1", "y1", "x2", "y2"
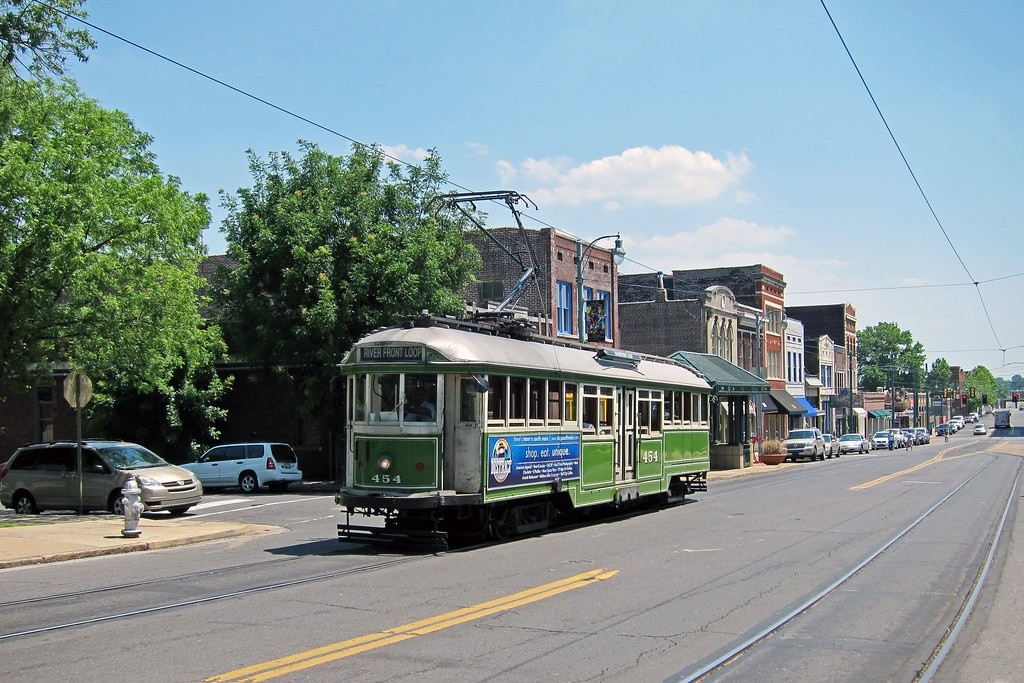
[{"x1": 337, "y1": 315, "x2": 715, "y2": 547}]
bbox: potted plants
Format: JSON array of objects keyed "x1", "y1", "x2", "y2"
[{"x1": 759, "y1": 441, "x2": 786, "y2": 466}]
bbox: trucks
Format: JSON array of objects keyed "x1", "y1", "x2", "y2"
[{"x1": 993, "y1": 409, "x2": 1011, "y2": 428}]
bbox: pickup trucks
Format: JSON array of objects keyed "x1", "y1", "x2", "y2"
[{"x1": 178, "y1": 443, "x2": 303, "y2": 496}]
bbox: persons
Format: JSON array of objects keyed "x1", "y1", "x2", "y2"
[
  {"x1": 887, "y1": 428, "x2": 895, "y2": 451},
  {"x1": 906, "y1": 430, "x2": 914, "y2": 451},
  {"x1": 944, "y1": 426, "x2": 949, "y2": 444},
  {"x1": 899, "y1": 432, "x2": 907, "y2": 448},
  {"x1": 406, "y1": 386, "x2": 436, "y2": 422}
]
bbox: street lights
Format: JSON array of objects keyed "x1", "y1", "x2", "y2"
[
  {"x1": 576, "y1": 232, "x2": 626, "y2": 345},
  {"x1": 891, "y1": 366, "x2": 909, "y2": 428}
]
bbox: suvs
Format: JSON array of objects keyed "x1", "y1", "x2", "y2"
[{"x1": 781, "y1": 427, "x2": 826, "y2": 462}]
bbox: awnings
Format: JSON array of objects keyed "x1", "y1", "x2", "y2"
[
  {"x1": 794, "y1": 398, "x2": 817, "y2": 416},
  {"x1": 770, "y1": 390, "x2": 808, "y2": 414},
  {"x1": 881, "y1": 409, "x2": 891, "y2": 416},
  {"x1": 874, "y1": 409, "x2": 886, "y2": 416},
  {"x1": 853, "y1": 407, "x2": 867, "y2": 416},
  {"x1": 868, "y1": 410, "x2": 880, "y2": 420},
  {"x1": 761, "y1": 394, "x2": 780, "y2": 413}
]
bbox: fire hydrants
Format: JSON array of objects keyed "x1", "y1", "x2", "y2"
[{"x1": 121, "y1": 478, "x2": 144, "y2": 538}]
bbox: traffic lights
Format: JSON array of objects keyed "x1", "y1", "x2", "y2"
[
  {"x1": 943, "y1": 388, "x2": 948, "y2": 398},
  {"x1": 1012, "y1": 393, "x2": 1016, "y2": 402},
  {"x1": 756, "y1": 308, "x2": 788, "y2": 461},
  {"x1": 962, "y1": 395, "x2": 967, "y2": 404},
  {"x1": 951, "y1": 389, "x2": 956, "y2": 398},
  {"x1": 970, "y1": 386, "x2": 975, "y2": 398},
  {"x1": 982, "y1": 395, "x2": 987, "y2": 404}
]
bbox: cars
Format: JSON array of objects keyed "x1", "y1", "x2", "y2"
[
  {"x1": 822, "y1": 434, "x2": 840, "y2": 458},
  {"x1": 973, "y1": 424, "x2": 986, "y2": 435},
  {"x1": 871, "y1": 431, "x2": 894, "y2": 450},
  {"x1": 936, "y1": 424, "x2": 952, "y2": 436},
  {"x1": 884, "y1": 428, "x2": 905, "y2": 448},
  {"x1": 838, "y1": 434, "x2": 870, "y2": 454},
  {"x1": 902, "y1": 428, "x2": 928, "y2": 446}
]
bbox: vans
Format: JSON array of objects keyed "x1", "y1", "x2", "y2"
[
  {"x1": 0, "y1": 437, "x2": 202, "y2": 516},
  {"x1": 950, "y1": 415, "x2": 965, "y2": 431}
]
